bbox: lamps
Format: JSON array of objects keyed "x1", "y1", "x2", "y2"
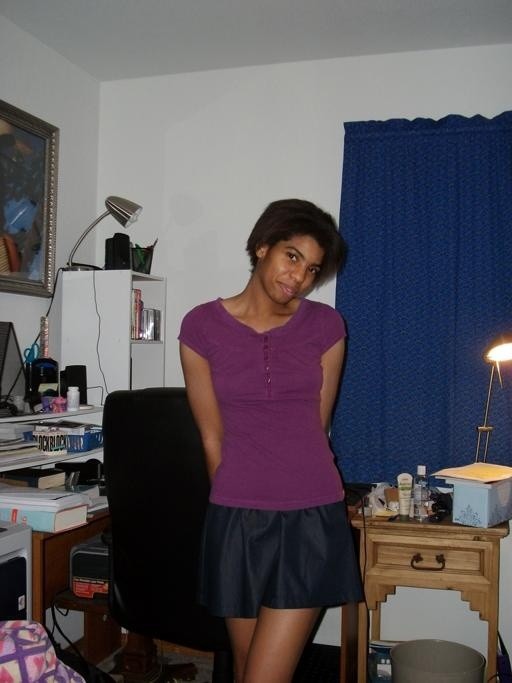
[
  {"x1": 61, "y1": 196, "x2": 142, "y2": 271},
  {"x1": 475, "y1": 332, "x2": 512, "y2": 462}
]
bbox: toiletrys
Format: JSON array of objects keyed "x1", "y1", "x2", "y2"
[{"x1": 396, "y1": 470, "x2": 412, "y2": 522}]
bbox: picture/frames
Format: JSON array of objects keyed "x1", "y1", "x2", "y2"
[{"x1": 1, "y1": 100, "x2": 59, "y2": 298}]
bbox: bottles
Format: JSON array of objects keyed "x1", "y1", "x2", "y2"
[
  {"x1": 412, "y1": 463, "x2": 430, "y2": 524},
  {"x1": 65, "y1": 386, "x2": 80, "y2": 412}
]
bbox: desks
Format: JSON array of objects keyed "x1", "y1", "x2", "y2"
[{"x1": 338, "y1": 485, "x2": 510, "y2": 683}]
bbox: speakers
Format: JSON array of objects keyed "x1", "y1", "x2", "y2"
[
  {"x1": 105, "y1": 232, "x2": 132, "y2": 271},
  {"x1": 59, "y1": 365, "x2": 89, "y2": 407}
]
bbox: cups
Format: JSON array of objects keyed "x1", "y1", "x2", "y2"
[{"x1": 129, "y1": 246, "x2": 153, "y2": 273}]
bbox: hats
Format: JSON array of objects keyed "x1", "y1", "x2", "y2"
[{"x1": 0, "y1": 620, "x2": 86, "y2": 683}]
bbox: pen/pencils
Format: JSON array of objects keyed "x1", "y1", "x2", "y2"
[{"x1": 135, "y1": 238, "x2": 159, "y2": 273}]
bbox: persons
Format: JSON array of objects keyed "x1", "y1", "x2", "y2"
[{"x1": 176, "y1": 198, "x2": 365, "y2": 681}]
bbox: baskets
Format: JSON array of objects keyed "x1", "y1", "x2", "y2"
[{"x1": 24, "y1": 430, "x2": 104, "y2": 453}]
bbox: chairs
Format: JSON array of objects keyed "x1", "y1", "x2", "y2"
[{"x1": 103, "y1": 387, "x2": 233, "y2": 682}]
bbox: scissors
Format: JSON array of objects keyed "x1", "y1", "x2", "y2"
[{"x1": 24, "y1": 343, "x2": 39, "y2": 362}]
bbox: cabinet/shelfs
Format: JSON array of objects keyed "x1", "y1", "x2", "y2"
[
  {"x1": 0, "y1": 408, "x2": 123, "y2": 664},
  {"x1": 60, "y1": 270, "x2": 167, "y2": 408}
]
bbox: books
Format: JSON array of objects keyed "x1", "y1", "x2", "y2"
[
  {"x1": 1, "y1": 422, "x2": 107, "y2": 531},
  {"x1": 131, "y1": 289, "x2": 160, "y2": 341}
]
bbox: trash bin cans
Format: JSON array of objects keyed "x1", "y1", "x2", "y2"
[{"x1": 389, "y1": 639, "x2": 485, "y2": 683}]
